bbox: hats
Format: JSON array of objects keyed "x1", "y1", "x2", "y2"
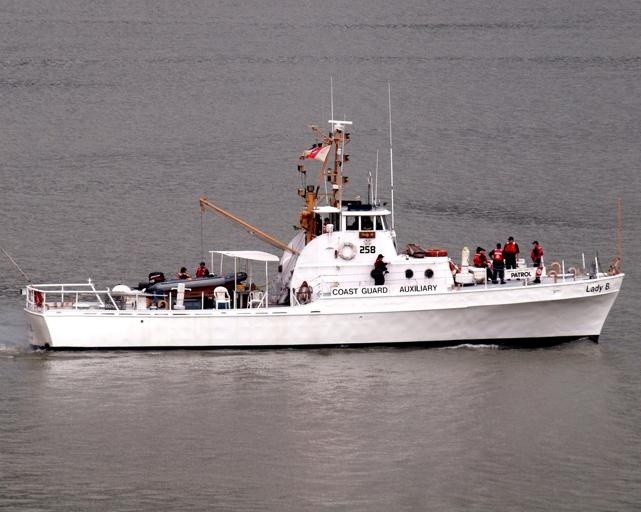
[
  {"x1": 200, "y1": 263, "x2": 205, "y2": 266},
  {"x1": 477, "y1": 247, "x2": 481, "y2": 253},
  {"x1": 378, "y1": 254, "x2": 383, "y2": 259},
  {"x1": 181, "y1": 267, "x2": 186, "y2": 272},
  {"x1": 507, "y1": 237, "x2": 513, "y2": 240},
  {"x1": 532, "y1": 241, "x2": 538, "y2": 245},
  {"x1": 497, "y1": 243, "x2": 501, "y2": 248}
]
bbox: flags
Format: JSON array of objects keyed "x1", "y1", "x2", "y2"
[{"x1": 301, "y1": 144, "x2": 331, "y2": 162}]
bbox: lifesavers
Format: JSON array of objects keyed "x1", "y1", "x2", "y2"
[{"x1": 338, "y1": 242, "x2": 357, "y2": 260}]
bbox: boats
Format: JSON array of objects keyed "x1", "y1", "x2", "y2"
[{"x1": 18, "y1": 76, "x2": 627, "y2": 351}]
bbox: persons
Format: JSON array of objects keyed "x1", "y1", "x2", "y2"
[
  {"x1": 502, "y1": 236, "x2": 521, "y2": 281},
  {"x1": 323, "y1": 218, "x2": 330, "y2": 233},
  {"x1": 374, "y1": 254, "x2": 391, "y2": 285},
  {"x1": 176, "y1": 266, "x2": 192, "y2": 280},
  {"x1": 315, "y1": 214, "x2": 322, "y2": 236},
  {"x1": 530, "y1": 240, "x2": 544, "y2": 284},
  {"x1": 195, "y1": 261, "x2": 209, "y2": 278},
  {"x1": 473, "y1": 242, "x2": 506, "y2": 284}
]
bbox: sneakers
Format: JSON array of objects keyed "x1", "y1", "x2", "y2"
[
  {"x1": 492, "y1": 280, "x2": 505, "y2": 284},
  {"x1": 533, "y1": 279, "x2": 540, "y2": 283}
]
